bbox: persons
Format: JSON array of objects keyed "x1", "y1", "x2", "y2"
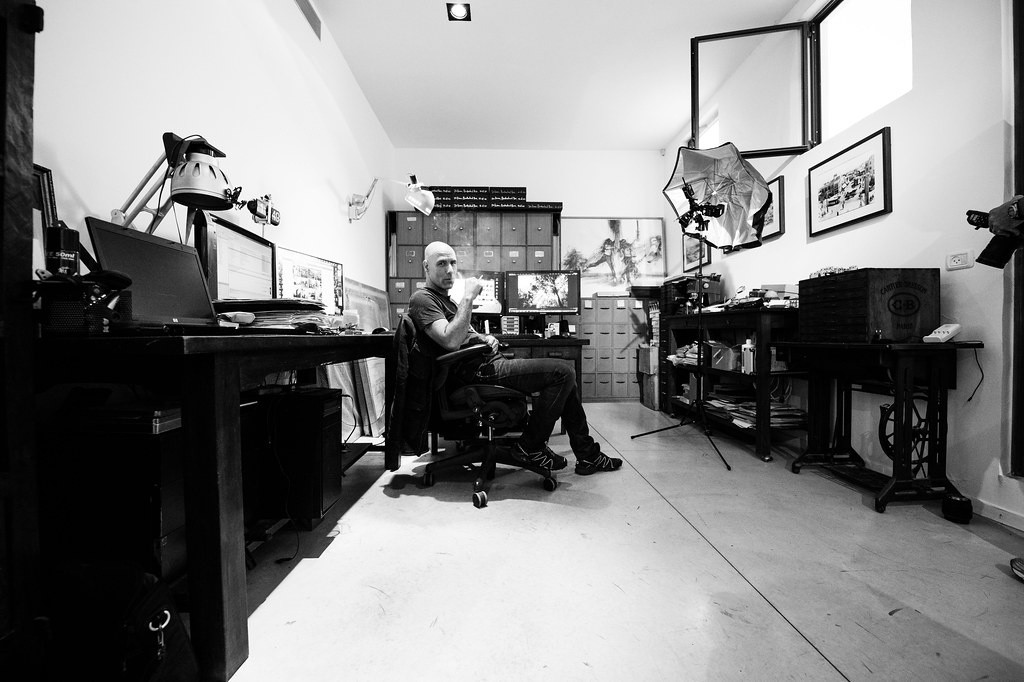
[
  {"x1": 409, "y1": 240, "x2": 623, "y2": 477},
  {"x1": 988, "y1": 193, "x2": 1024, "y2": 580}
]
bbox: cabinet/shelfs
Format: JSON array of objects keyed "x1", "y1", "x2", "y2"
[
  {"x1": 662, "y1": 305, "x2": 819, "y2": 462},
  {"x1": 385, "y1": 209, "x2": 552, "y2": 334}
]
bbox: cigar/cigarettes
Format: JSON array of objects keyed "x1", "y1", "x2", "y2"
[{"x1": 477, "y1": 275, "x2": 483, "y2": 280}]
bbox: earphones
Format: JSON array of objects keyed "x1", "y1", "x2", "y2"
[{"x1": 425, "y1": 264, "x2": 428, "y2": 270}]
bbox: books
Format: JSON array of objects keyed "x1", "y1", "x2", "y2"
[
  {"x1": 707, "y1": 385, "x2": 757, "y2": 411},
  {"x1": 731, "y1": 400, "x2": 806, "y2": 428}
]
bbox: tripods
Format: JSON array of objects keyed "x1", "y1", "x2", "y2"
[{"x1": 630, "y1": 233, "x2": 732, "y2": 471}]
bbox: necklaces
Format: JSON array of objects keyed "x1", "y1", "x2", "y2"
[{"x1": 425, "y1": 288, "x2": 473, "y2": 331}]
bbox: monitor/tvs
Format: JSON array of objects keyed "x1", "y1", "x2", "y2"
[
  {"x1": 208, "y1": 213, "x2": 277, "y2": 300},
  {"x1": 448, "y1": 270, "x2": 582, "y2": 335}
]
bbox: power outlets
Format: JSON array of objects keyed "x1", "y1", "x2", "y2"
[
  {"x1": 945, "y1": 250, "x2": 974, "y2": 271},
  {"x1": 962, "y1": 380, "x2": 981, "y2": 405}
]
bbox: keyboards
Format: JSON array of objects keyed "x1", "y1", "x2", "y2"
[{"x1": 485, "y1": 333, "x2": 540, "y2": 339}]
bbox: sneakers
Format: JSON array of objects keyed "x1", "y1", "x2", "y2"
[
  {"x1": 510, "y1": 442, "x2": 568, "y2": 471},
  {"x1": 575, "y1": 452, "x2": 622, "y2": 475}
]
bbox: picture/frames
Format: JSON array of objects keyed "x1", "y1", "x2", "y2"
[
  {"x1": 682, "y1": 232, "x2": 711, "y2": 273},
  {"x1": 807, "y1": 126, "x2": 893, "y2": 236},
  {"x1": 31, "y1": 161, "x2": 60, "y2": 309},
  {"x1": 760, "y1": 175, "x2": 786, "y2": 240}
]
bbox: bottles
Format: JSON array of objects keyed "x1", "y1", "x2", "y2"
[
  {"x1": 44, "y1": 227, "x2": 79, "y2": 278},
  {"x1": 342, "y1": 309, "x2": 360, "y2": 329},
  {"x1": 742, "y1": 339, "x2": 754, "y2": 373}
]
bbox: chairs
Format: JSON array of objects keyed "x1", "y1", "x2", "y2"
[{"x1": 395, "y1": 309, "x2": 558, "y2": 506}]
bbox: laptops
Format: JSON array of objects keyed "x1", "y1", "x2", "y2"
[{"x1": 85, "y1": 217, "x2": 306, "y2": 336}]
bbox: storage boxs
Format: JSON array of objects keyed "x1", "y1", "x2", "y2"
[{"x1": 638, "y1": 343, "x2": 660, "y2": 412}]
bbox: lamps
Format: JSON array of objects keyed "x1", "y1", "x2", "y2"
[
  {"x1": 347, "y1": 174, "x2": 437, "y2": 217},
  {"x1": 110, "y1": 131, "x2": 233, "y2": 233}
]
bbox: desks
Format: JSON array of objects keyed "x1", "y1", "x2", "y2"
[
  {"x1": 47, "y1": 325, "x2": 395, "y2": 680},
  {"x1": 476, "y1": 335, "x2": 590, "y2": 434},
  {"x1": 776, "y1": 339, "x2": 987, "y2": 524}
]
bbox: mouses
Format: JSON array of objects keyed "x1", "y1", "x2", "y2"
[
  {"x1": 372, "y1": 327, "x2": 389, "y2": 334},
  {"x1": 562, "y1": 332, "x2": 570, "y2": 338}
]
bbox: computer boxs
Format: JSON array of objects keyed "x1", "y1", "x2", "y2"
[{"x1": 240, "y1": 384, "x2": 343, "y2": 515}]
